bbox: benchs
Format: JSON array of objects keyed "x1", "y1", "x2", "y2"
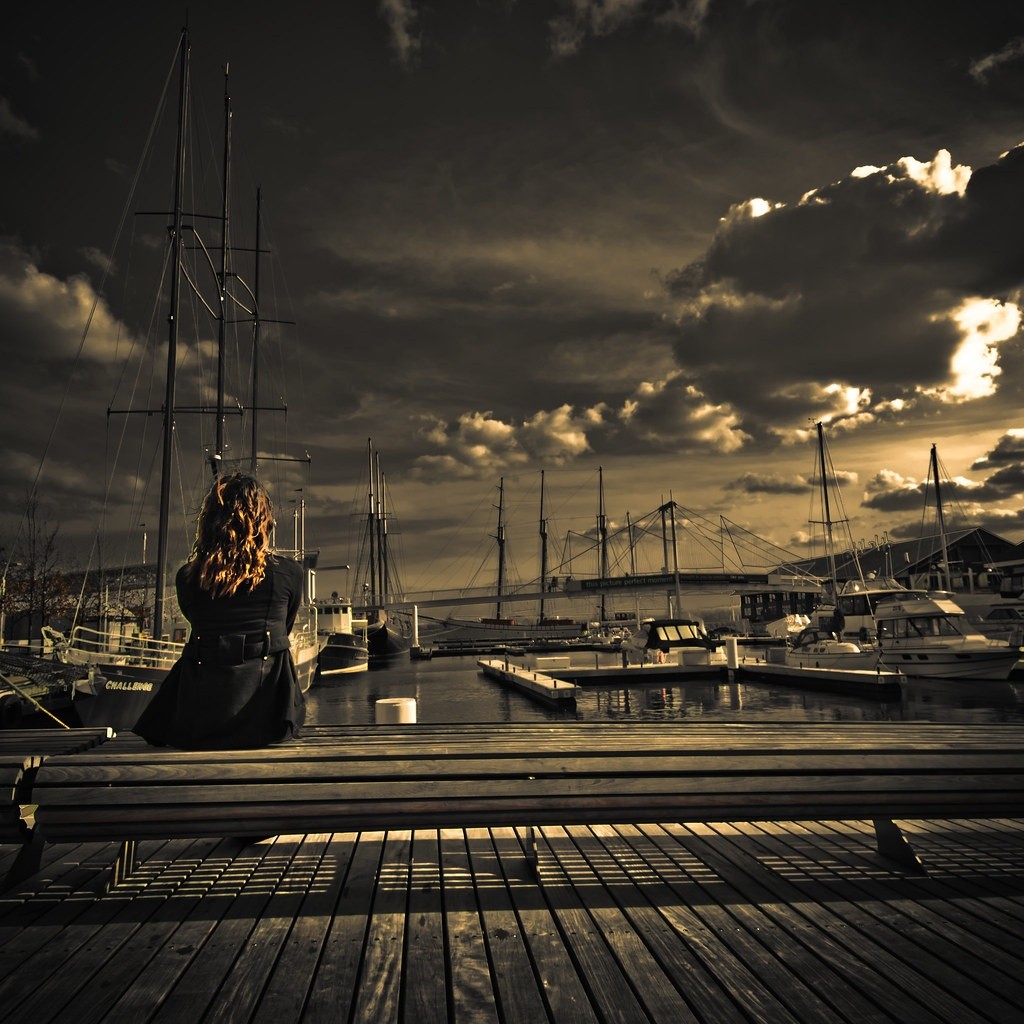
[
  {"x1": 0, "y1": 727, "x2": 118, "y2": 844},
  {"x1": 4, "y1": 721, "x2": 1024, "y2": 902}
]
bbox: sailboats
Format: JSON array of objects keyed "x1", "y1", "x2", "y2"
[
  {"x1": 785, "y1": 419, "x2": 1023, "y2": 681},
  {"x1": 390, "y1": 465, "x2": 656, "y2": 646},
  {"x1": 810, "y1": 442, "x2": 1023, "y2": 670},
  {"x1": 1, "y1": 6, "x2": 320, "y2": 731},
  {"x1": 344, "y1": 436, "x2": 413, "y2": 657}
]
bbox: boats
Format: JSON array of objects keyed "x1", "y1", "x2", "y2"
[
  {"x1": 619, "y1": 616, "x2": 728, "y2": 663},
  {"x1": 287, "y1": 489, "x2": 368, "y2": 678}
]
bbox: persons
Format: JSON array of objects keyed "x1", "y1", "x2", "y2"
[{"x1": 132, "y1": 474, "x2": 307, "y2": 751}]
